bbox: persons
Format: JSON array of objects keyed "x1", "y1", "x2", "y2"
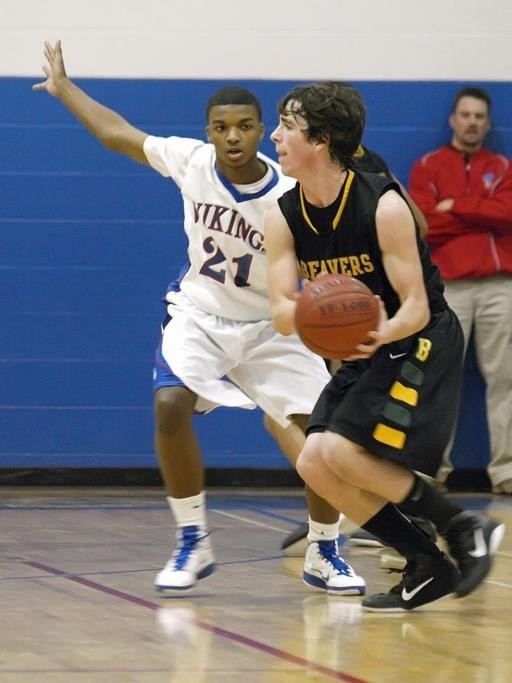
[
  {"x1": 262, "y1": 79, "x2": 509, "y2": 615},
  {"x1": 28, "y1": 37, "x2": 370, "y2": 604},
  {"x1": 261, "y1": 144, "x2": 428, "y2": 555},
  {"x1": 407, "y1": 87, "x2": 512, "y2": 497}
]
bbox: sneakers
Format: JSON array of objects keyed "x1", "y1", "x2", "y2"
[
  {"x1": 360, "y1": 549, "x2": 465, "y2": 616},
  {"x1": 155, "y1": 602, "x2": 217, "y2": 661},
  {"x1": 347, "y1": 524, "x2": 391, "y2": 548},
  {"x1": 278, "y1": 521, "x2": 313, "y2": 559},
  {"x1": 487, "y1": 471, "x2": 511, "y2": 498},
  {"x1": 434, "y1": 507, "x2": 509, "y2": 602},
  {"x1": 151, "y1": 521, "x2": 220, "y2": 600},
  {"x1": 298, "y1": 537, "x2": 368, "y2": 599},
  {"x1": 303, "y1": 598, "x2": 367, "y2": 641}
]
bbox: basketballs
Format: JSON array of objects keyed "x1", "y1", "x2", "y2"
[{"x1": 296, "y1": 276, "x2": 379, "y2": 360}]
